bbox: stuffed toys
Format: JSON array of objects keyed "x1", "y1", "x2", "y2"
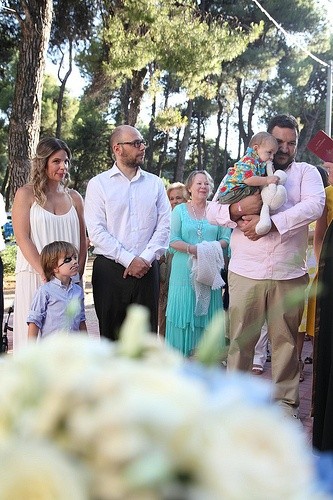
[{"x1": 255, "y1": 158, "x2": 288, "y2": 236}]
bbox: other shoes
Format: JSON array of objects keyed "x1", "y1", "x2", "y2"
[
  {"x1": 297, "y1": 359, "x2": 304, "y2": 382},
  {"x1": 304, "y1": 356, "x2": 312, "y2": 364},
  {"x1": 251, "y1": 364, "x2": 264, "y2": 374}
]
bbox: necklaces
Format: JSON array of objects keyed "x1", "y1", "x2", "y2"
[{"x1": 190, "y1": 201, "x2": 208, "y2": 240}]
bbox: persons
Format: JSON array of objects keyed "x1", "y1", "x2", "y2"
[
  {"x1": 25, "y1": 240, "x2": 89, "y2": 338},
  {"x1": 164, "y1": 170, "x2": 232, "y2": 362},
  {"x1": 303, "y1": 335, "x2": 314, "y2": 365},
  {"x1": 206, "y1": 114, "x2": 326, "y2": 419},
  {"x1": 297, "y1": 162, "x2": 333, "y2": 382},
  {"x1": 158, "y1": 182, "x2": 190, "y2": 341},
  {"x1": 84, "y1": 125, "x2": 171, "y2": 341},
  {"x1": 220, "y1": 259, "x2": 272, "y2": 375},
  {"x1": 12, "y1": 137, "x2": 88, "y2": 350},
  {"x1": 218, "y1": 131, "x2": 280, "y2": 226}
]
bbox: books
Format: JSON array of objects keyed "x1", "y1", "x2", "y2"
[{"x1": 306, "y1": 129, "x2": 333, "y2": 163}]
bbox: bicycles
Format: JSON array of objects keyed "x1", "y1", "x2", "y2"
[{"x1": 1, "y1": 306, "x2": 20, "y2": 352}]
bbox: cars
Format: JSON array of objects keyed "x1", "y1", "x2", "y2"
[{"x1": 1, "y1": 216, "x2": 15, "y2": 243}]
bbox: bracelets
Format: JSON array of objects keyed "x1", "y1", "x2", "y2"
[
  {"x1": 186, "y1": 244, "x2": 190, "y2": 254},
  {"x1": 237, "y1": 201, "x2": 245, "y2": 217}
]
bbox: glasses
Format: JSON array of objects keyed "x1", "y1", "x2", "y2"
[{"x1": 118, "y1": 138, "x2": 150, "y2": 149}]
